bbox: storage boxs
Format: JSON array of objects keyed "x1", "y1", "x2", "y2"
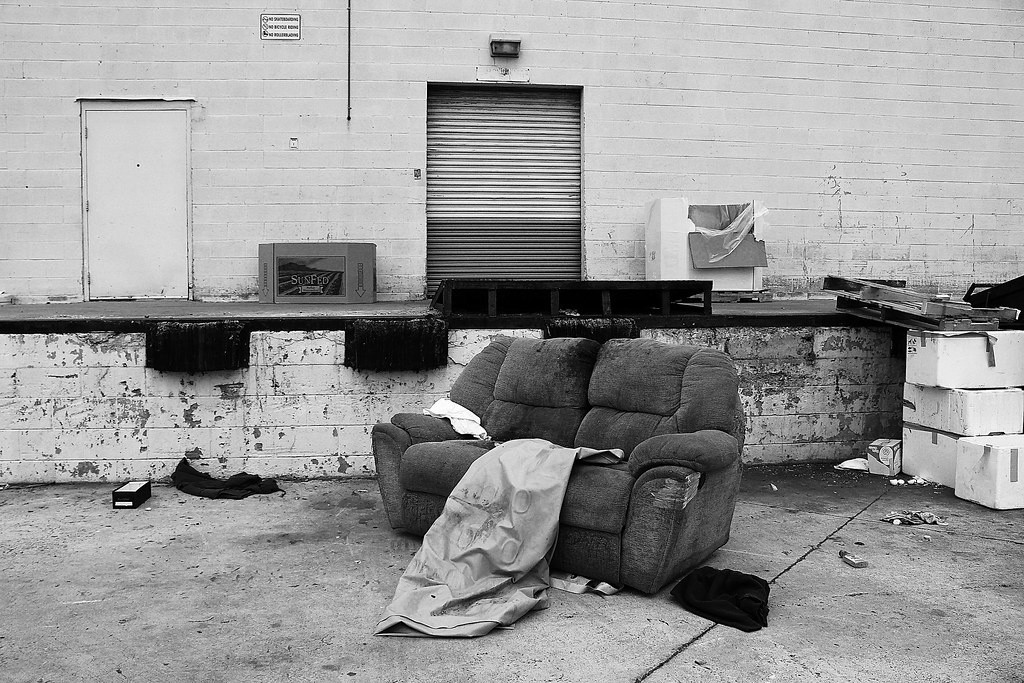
[
  {"x1": 867, "y1": 438, "x2": 902, "y2": 476},
  {"x1": 112, "y1": 480, "x2": 152, "y2": 510},
  {"x1": 258, "y1": 242, "x2": 377, "y2": 304},
  {"x1": 644, "y1": 197, "x2": 768, "y2": 292},
  {"x1": 900, "y1": 326, "x2": 1024, "y2": 511}
]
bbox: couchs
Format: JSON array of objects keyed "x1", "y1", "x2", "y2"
[{"x1": 370, "y1": 333, "x2": 746, "y2": 596}]
